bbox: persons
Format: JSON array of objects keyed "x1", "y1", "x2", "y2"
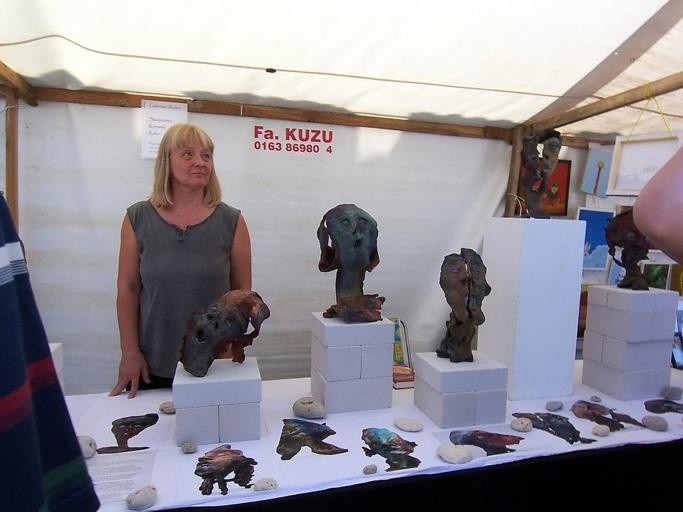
[
  {"x1": 107, "y1": 123, "x2": 251, "y2": 399},
  {"x1": 633, "y1": 145, "x2": 683, "y2": 267}
]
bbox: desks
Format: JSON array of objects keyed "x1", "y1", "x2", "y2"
[{"x1": 55, "y1": 358, "x2": 683, "y2": 512}]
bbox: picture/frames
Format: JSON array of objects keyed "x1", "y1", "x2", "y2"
[
  {"x1": 515, "y1": 159, "x2": 572, "y2": 216},
  {"x1": 576, "y1": 206, "x2": 616, "y2": 270},
  {"x1": 672, "y1": 332, "x2": 683, "y2": 368}
]
